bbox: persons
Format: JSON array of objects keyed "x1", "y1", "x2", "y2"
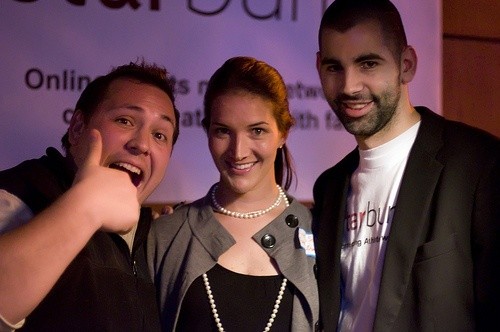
[
  {"x1": 146, "y1": 55, "x2": 320, "y2": 332},
  {"x1": 0, "y1": 61, "x2": 186, "y2": 332},
  {"x1": 314, "y1": 0, "x2": 499, "y2": 331}
]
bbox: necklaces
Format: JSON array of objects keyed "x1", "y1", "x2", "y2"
[{"x1": 201, "y1": 178, "x2": 293, "y2": 332}]
